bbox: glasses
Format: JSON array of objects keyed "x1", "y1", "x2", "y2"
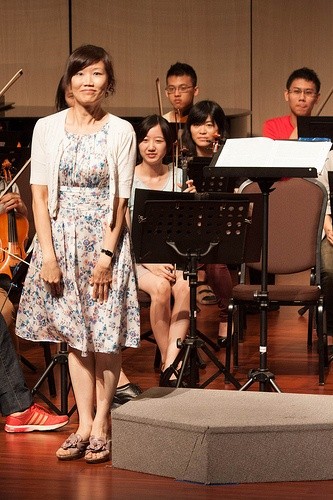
[
  {"x1": 288, "y1": 88, "x2": 319, "y2": 96},
  {"x1": 165, "y1": 85, "x2": 195, "y2": 93}
]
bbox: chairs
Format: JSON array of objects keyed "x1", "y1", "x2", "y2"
[{"x1": 224, "y1": 178, "x2": 328, "y2": 386}]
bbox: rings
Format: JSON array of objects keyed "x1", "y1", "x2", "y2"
[{"x1": 14, "y1": 199, "x2": 20, "y2": 204}]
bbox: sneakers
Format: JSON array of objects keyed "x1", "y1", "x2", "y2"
[{"x1": 4, "y1": 402, "x2": 69, "y2": 432}]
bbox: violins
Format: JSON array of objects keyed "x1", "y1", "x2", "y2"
[{"x1": 0, "y1": 159, "x2": 29, "y2": 267}]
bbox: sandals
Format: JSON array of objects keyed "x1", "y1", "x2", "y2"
[
  {"x1": 85, "y1": 434, "x2": 112, "y2": 463},
  {"x1": 55, "y1": 433, "x2": 89, "y2": 460}
]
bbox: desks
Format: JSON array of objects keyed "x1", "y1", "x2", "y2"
[{"x1": 0, "y1": 106, "x2": 251, "y2": 156}]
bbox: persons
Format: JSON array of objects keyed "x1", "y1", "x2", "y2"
[
  {"x1": 153, "y1": 62, "x2": 220, "y2": 306},
  {"x1": 125, "y1": 114, "x2": 194, "y2": 389},
  {"x1": 54, "y1": 71, "x2": 147, "y2": 403},
  {"x1": 166, "y1": 100, "x2": 266, "y2": 347},
  {"x1": 0, "y1": 175, "x2": 71, "y2": 433},
  {"x1": 261, "y1": 65, "x2": 333, "y2": 364},
  {"x1": 14, "y1": 43, "x2": 143, "y2": 464}
]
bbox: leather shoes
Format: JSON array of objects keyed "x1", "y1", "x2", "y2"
[
  {"x1": 218, "y1": 318, "x2": 236, "y2": 347},
  {"x1": 111, "y1": 383, "x2": 144, "y2": 407},
  {"x1": 161, "y1": 363, "x2": 183, "y2": 388}
]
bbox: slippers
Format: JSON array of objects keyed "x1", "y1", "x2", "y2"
[{"x1": 196, "y1": 283, "x2": 219, "y2": 305}]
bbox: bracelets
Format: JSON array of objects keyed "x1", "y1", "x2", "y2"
[{"x1": 100, "y1": 248, "x2": 116, "y2": 260}]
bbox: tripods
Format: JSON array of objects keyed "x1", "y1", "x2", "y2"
[{"x1": 17, "y1": 137, "x2": 332, "y2": 417}]
